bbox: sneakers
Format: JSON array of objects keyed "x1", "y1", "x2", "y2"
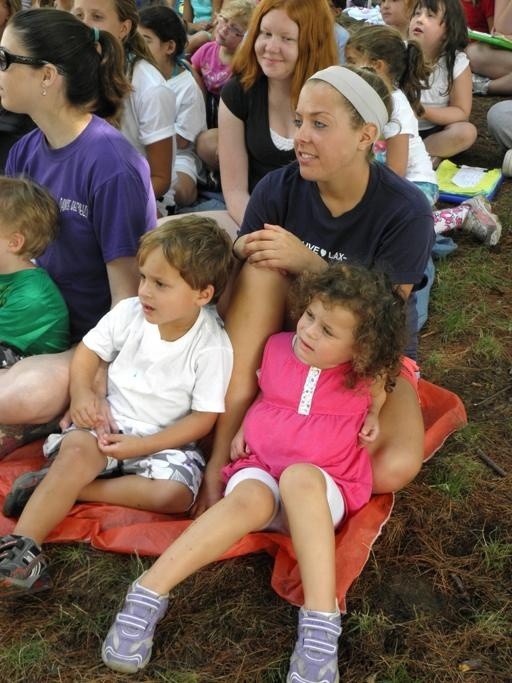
[
  {"x1": 461, "y1": 194, "x2": 502, "y2": 249},
  {"x1": 286, "y1": 598, "x2": 343, "y2": 682},
  {"x1": 101, "y1": 569, "x2": 170, "y2": 672},
  {"x1": 0, "y1": 532, "x2": 54, "y2": 598},
  {"x1": 4, "y1": 449, "x2": 57, "y2": 517}
]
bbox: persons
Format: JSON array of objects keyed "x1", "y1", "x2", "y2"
[
  {"x1": 1, "y1": 0, "x2": 512, "y2": 496},
  {"x1": 0, "y1": 213, "x2": 242, "y2": 601},
  {"x1": 99, "y1": 261, "x2": 407, "y2": 681}
]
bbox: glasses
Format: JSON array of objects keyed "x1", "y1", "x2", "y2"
[
  {"x1": 0, "y1": 49, "x2": 65, "y2": 76},
  {"x1": 216, "y1": 14, "x2": 244, "y2": 37}
]
